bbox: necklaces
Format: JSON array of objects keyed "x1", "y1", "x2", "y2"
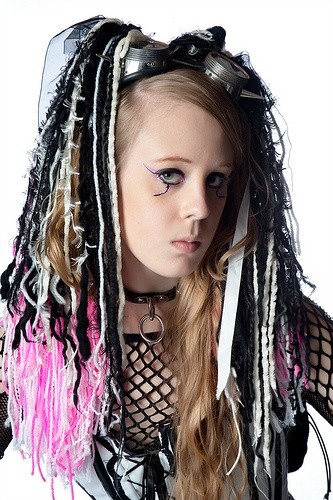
[{"x1": 122, "y1": 282, "x2": 178, "y2": 345}]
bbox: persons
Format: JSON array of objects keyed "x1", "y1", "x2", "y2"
[{"x1": 0, "y1": 14, "x2": 333, "y2": 499}]
